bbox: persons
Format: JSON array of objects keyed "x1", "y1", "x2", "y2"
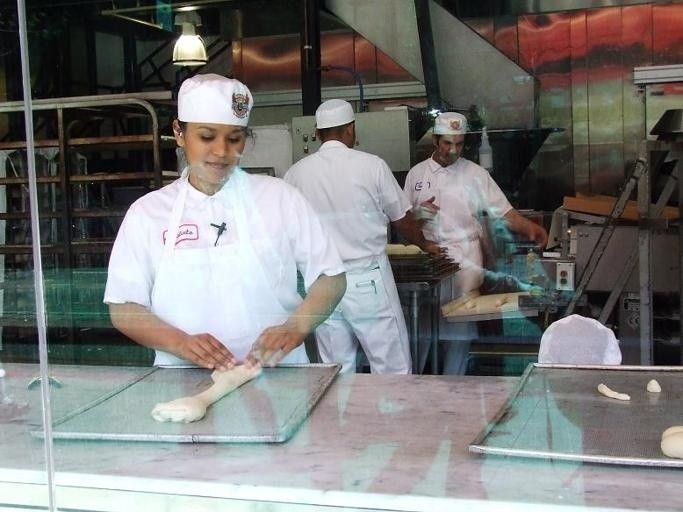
[
  {"x1": 403, "y1": 109, "x2": 550, "y2": 376},
  {"x1": 103, "y1": 71, "x2": 347, "y2": 373},
  {"x1": 284, "y1": 98, "x2": 443, "y2": 377}
]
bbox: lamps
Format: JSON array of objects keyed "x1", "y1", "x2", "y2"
[{"x1": 171, "y1": 10, "x2": 206, "y2": 68}]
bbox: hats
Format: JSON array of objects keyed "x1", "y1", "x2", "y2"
[
  {"x1": 177, "y1": 73, "x2": 257, "y2": 128},
  {"x1": 433, "y1": 111, "x2": 469, "y2": 136},
  {"x1": 314, "y1": 98, "x2": 356, "y2": 130}
]
